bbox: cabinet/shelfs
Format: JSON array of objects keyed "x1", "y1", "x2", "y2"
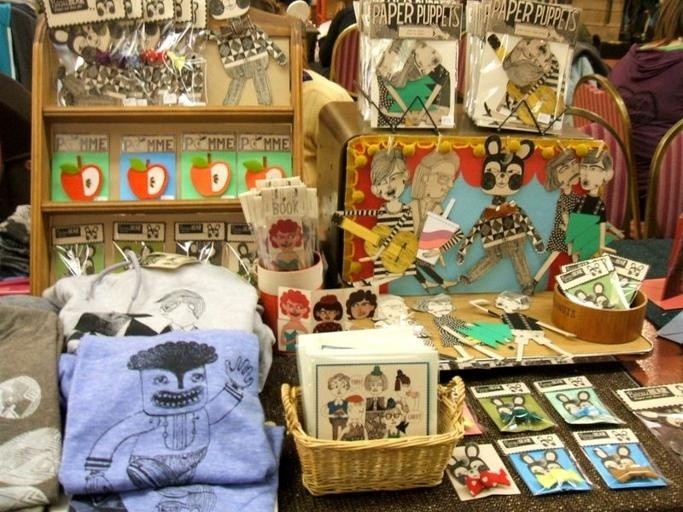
[{"x1": 30, "y1": 7, "x2": 306, "y2": 297}]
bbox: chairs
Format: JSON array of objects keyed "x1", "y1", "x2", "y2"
[
  {"x1": 563, "y1": 74, "x2": 682, "y2": 239},
  {"x1": 330, "y1": 22, "x2": 359, "y2": 99}
]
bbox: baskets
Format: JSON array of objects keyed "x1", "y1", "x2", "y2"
[{"x1": 278, "y1": 375, "x2": 467, "y2": 498}]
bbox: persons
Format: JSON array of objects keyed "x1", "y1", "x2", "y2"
[
  {"x1": 531, "y1": 148, "x2": 624, "y2": 296},
  {"x1": 268, "y1": 220, "x2": 305, "y2": 271},
  {"x1": 280, "y1": 288, "x2": 377, "y2": 351},
  {"x1": 410, "y1": 290, "x2": 576, "y2": 362},
  {"x1": 606, "y1": 0, "x2": 683, "y2": 222},
  {"x1": 320, "y1": 364, "x2": 419, "y2": 440},
  {"x1": 336, "y1": 142, "x2": 460, "y2": 293}
]
